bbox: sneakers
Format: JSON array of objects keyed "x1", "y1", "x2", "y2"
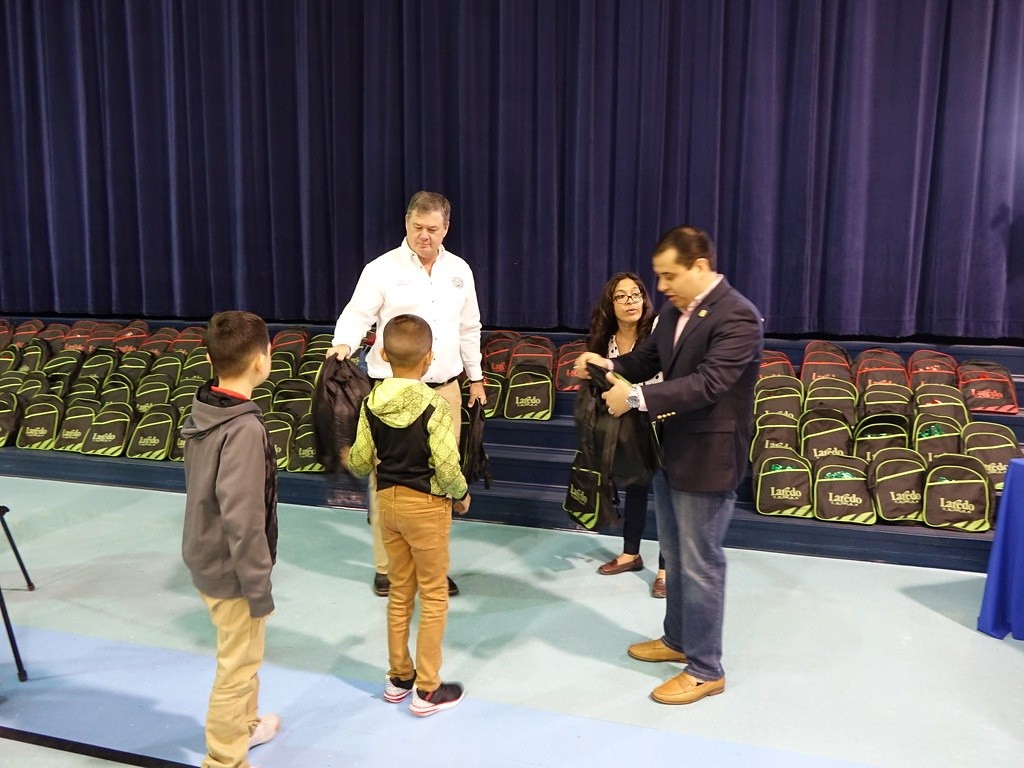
[
  {"x1": 248, "y1": 714, "x2": 280, "y2": 750},
  {"x1": 383, "y1": 669, "x2": 416, "y2": 703},
  {"x1": 409, "y1": 683, "x2": 465, "y2": 716}
]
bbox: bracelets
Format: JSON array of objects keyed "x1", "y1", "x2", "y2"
[{"x1": 468, "y1": 379, "x2": 485, "y2": 383}]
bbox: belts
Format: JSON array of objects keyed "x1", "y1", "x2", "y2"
[{"x1": 367, "y1": 376, "x2": 462, "y2": 389}]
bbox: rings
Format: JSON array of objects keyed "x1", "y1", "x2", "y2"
[{"x1": 608, "y1": 407, "x2": 614, "y2": 415}]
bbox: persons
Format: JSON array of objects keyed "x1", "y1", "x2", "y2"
[
  {"x1": 340, "y1": 314, "x2": 471, "y2": 717},
  {"x1": 573, "y1": 224, "x2": 764, "y2": 705},
  {"x1": 325, "y1": 191, "x2": 487, "y2": 597},
  {"x1": 587, "y1": 270, "x2": 666, "y2": 599},
  {"x1": 180, "y1": 310, "x2": 278, "y2": 768}
]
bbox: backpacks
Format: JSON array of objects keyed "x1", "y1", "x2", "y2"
[
  {"x1": 250, "y1": 328, "x2": 376, "y2": 489},
  {"x1": 563, "y1": 362, "x2": 663, "y2": 532},
  {"x1": 459, "y1": 330, "x2": 588, "y2": 421},
  {"x1": 458, "y1": 390, "x2": 489, "y2": 489},
  {"x1": 0, "y1": 318, "x2": 216, "y2": 461},
  {"x1": 748, "y1": 340, "x2": 1024, "y2": 533}
]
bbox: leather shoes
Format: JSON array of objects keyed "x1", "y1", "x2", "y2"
[
  {"x1": 375, "y1": 573, "x2": 390, "y2": 596},
  {"x1": 653, "y1": 578, "x2": 666, "y2": 599},
  {"x1": 651, "y1": 669, "x2": 725, "y2": 705},
  {"x1": 599, "y1": 554, "x2": 644, "y2": 575},
  {"x1": 627, "y1": 638, "x2": 689, "y2": 663},
  {"x1": 447, "y1": 576, "x2": 458, "y2": 595}
]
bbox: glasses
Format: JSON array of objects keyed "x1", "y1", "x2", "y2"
[{"x1": 614, "y1": 292, "x2": 643, "y2": 303}]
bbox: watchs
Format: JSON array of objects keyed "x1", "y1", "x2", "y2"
[{"x1": 625, "y1": 388, "x2": 641, "y2": 409}]
